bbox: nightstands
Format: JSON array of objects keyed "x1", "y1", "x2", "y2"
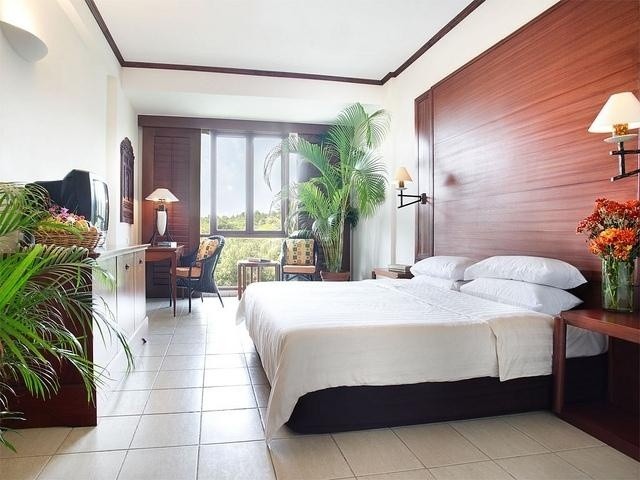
[
  {"x1": 544, "y1": 297, "x2": 640, "y2": 465},
  {"x1": 369, "y1": 267, "x2": 418, "y2": 280}
]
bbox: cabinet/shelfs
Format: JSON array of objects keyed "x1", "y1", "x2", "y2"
[
  {"x1": 134, "y1": 241, "x2": 151, "y2": 361},
  {"x1": 3, "y1": 246, "x2": 116, "y2": 430},
  {"x1": 116, "y1": 247, "x2": 134, "y2": 381}
]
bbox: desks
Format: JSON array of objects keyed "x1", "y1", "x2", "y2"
[
  {"x1": 235, "y1": 258, "x2": 281, "y2": 301},
  {"x1": 144, "y1": 241, "x2": 187, "y2": 318}
]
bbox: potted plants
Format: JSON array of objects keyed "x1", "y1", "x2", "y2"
[{"x1": 260, "y1": 99, "x2": 391, "y2": 282}]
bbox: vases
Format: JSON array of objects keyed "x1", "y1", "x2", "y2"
[{"x1": 597, "y1": 256, "x2": 638, "y2": 314}]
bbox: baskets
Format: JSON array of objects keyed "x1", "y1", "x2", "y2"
[{"x1": 34, "y1": 230, "x2": 102, "y2": 251}]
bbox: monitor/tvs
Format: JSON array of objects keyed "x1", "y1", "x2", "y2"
[{"x1": 25, "y1": 169, "x2": 110, "y2": 247}]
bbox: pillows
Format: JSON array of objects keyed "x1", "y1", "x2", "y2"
[
  {"x1": 407, "y1": 253, "x2": 481, "y2": 282},
  {"x1": 459, "y1": 274, "x2": 584, "y2": 315},
  {"x1": 193, "y1": 236, "x2": 220, "y2": 270},
  {"x1": 410, "y1": 272, "x2": 466, "y2": 292},
  {"x1": 459, "y1": 250, "x2": 593, "y2": 290},
  {"x1": 284, "y1": 237, "x2": 316, "y2": 267}
]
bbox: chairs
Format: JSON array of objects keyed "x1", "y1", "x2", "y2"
[
  {"x1": 276, "y1": 228, "x2": 324, "y2": 282},
  {"x1": 167, "y1": 232, "x2": 227, "y2": 315}
]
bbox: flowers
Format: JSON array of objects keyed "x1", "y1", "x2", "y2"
[
  {"x1": 33, "y1": 206, "x2": 94, "y2": 236},
  {"x1": 575, "y1": 195, "x2": 640, "y2": 308}
]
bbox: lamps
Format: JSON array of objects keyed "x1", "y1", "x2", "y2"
[
  {"x1": 144, "y1": 186, "x2": 180, "y2": 249},
  {"x1": 585, "y1": 88, "x2": 640, "y2": 183},
  {"x1": 389, "y1": 164, "x2": 429, "y2": 211}
]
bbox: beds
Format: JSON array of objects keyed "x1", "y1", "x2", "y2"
[{"x1": 233, "y1": 276, "x2": 615, "y2": 435}]
